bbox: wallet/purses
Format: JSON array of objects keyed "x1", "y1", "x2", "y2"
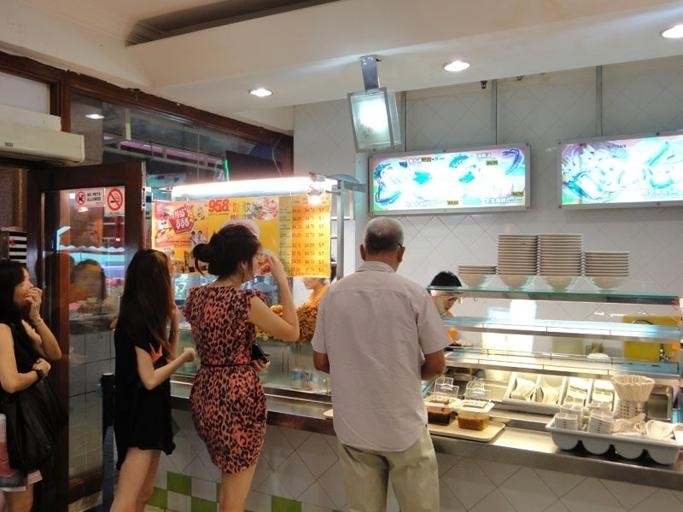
[{"x1": 251, "y1": 342, "x2": 269, "y2": 363}]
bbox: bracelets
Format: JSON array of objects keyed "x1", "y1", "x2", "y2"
[{"x1": 33, "y1": 319, "x2": 43, "y2": 325}]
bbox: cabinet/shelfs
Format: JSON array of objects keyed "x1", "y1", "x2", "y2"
[{"x1": 99, "y1": 274, "x2": 683, "y2": 511}]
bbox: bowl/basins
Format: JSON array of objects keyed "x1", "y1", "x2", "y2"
[{"x1": 459, "y1": 233, "x2": 628, "y2": 291}]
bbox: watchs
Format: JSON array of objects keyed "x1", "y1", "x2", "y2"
[{"x1": 31, "y1": 368, "x2": 45, "y2": 382}]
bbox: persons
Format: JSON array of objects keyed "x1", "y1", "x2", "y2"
[
  {"x1": 190, "y1": 230, "x2": 205, "y2": 248},
  {"x1": 71, "y1": 259, "x2": 105, "y2": 300},
  {"x1": 182, "y1": 225, "x2": 300, "y2": 512},
  {"x1": 0, "y1": 261, "x2": 62, "y2": 512},
  {"x1": 109, "y1": 249, "x2": 196, "y2": 512},
  {"x1": 304, "y1": 277, "x2": 329, "y2": 302},
  {"x1": 310, "y1": 216, "x2": 453, "y2": 512},
  {"x1": 426, "y1": 271, "x2": 462, "y2": 344}
]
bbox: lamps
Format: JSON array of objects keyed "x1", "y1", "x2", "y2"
[{"x1": 347, "y1": 52, "x2": 403, "y2": 154}]
